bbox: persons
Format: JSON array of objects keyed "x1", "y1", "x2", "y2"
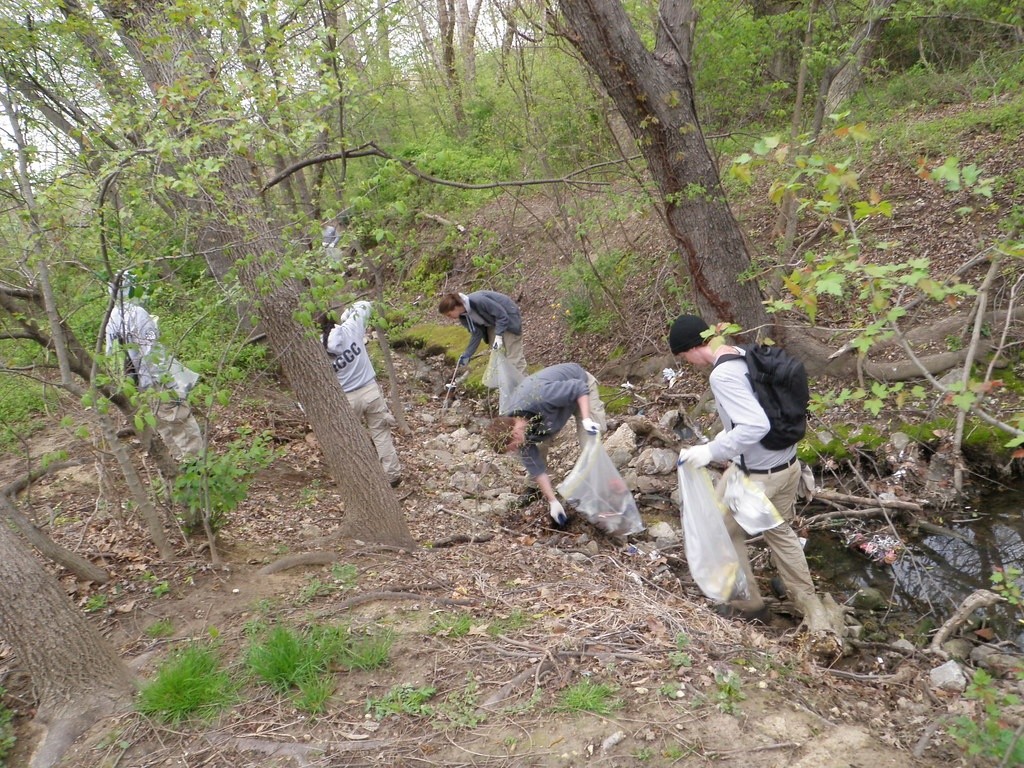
[
  {"x1": 103, "y1": 270, "x2": 208, "y2": 468},
  {"x1": 318, "y1": 301, "x2": 402, "y2": 488},
  {"x1": 485, "y1": 361, "x2": 609, "y2": 530},
  {"x1": 318, "y1": 217, "x2": 347, "y2": 270},
  {"x1": 439, "y1": 289, "x2": 530, "y2": 377},
  {"x1": 668, "y1": 313, "x2": 832, "y2": 635}
]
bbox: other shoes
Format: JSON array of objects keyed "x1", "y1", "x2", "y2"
[
  {"x1": 769, "y1": 575, "x2": 788, "y2": 602},
  {"x1": 709, "y1": 603, "x2": 771, "y2": 624},
  {"x1": 390, "y1": 477, "x2": 403, "y2": 488},
  {"x1": 516, "y1": 488, "x2": 541, "y2": 507}
]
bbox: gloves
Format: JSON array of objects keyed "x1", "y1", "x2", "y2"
[
  {"x1": 493, "y1": 335, "x2": 503, "y2": 350},
  {"x1": 677, "y1": 444, "x2": 713, "y2": 469},
  {"x1": 459, "y1": 355, "x2": 470, "y2": 366},
  {"x1": 581, "y1": 418, "x2": 600, "y2": 435},
  {"x1": 549, "y1": 499, "x2": 567, "y2": 526}
]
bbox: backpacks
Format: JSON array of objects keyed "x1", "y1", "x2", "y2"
[
  {"x1": 712, "y1": 343, "x2": 809, "y2": 475},
  {"x1": 110, "y1": 333, "x2": 139, "y2": 385}
]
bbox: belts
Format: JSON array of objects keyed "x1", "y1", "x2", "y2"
[{"x1": 729, "y1": 454, "x2": 796, "y2": 475}]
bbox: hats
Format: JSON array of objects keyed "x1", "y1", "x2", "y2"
[{"x1": 669, "y1": 315, "x2": 710, "y2": 356}]
